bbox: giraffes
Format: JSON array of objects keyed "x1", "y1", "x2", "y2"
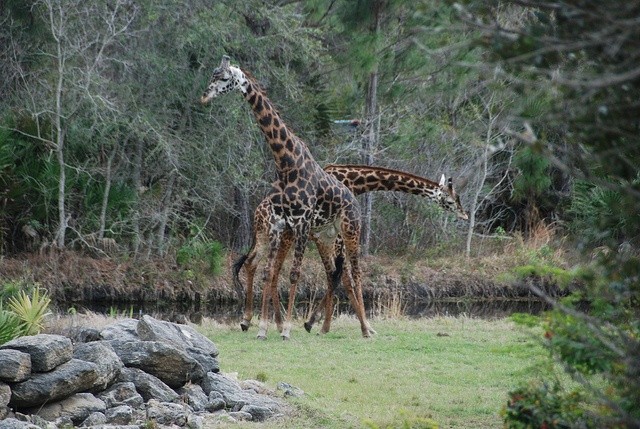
[
  {"x1": 231, "y1": 162, "x2": 469, "y2": 334},
  {"x1": 198, "y1": 55, "x2": 373, "y2": 341}
]
